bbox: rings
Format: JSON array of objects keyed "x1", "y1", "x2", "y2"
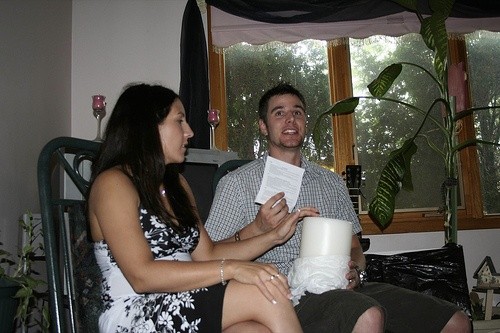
[
  {"x1": 274, "y1": 272, "x2": 280, "y2": 277},
  {"x1": 270, "y1": 275, "x2": 275, "y2": 282}
]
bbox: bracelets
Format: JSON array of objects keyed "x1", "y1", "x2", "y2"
[
  {"x1": 235, "y1": 231, "x2": 240, "y2": 242},
  {"x1": 220, "y1": 258, "x2": 227, "y2": 286}
]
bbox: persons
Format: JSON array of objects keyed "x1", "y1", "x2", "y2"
[
  {"x1": 203, "y1": 81, "x2": 475, "y2": 333},
  {"x1": 86, "y1": 83, "x2": 320, "y2": 333}
]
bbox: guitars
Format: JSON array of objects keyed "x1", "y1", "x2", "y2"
[{"x1": 342, "y1": 165, "x2": 367, "y2": 237}]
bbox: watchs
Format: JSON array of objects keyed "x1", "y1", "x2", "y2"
[{"x1": 355, "y1": 267, "x2": 369, "y2": 287}]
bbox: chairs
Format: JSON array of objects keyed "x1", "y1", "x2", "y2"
[{"x1": 38, "y1": 137, "x2": 100, "y2": 333}]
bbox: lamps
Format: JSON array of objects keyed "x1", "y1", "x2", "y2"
[
  {"x1": 206, "y1": 110, "x2": 220, "y2": 150},
  {"x1": 91, "y1": 95, "x2": 106, "y2": 142}
]
